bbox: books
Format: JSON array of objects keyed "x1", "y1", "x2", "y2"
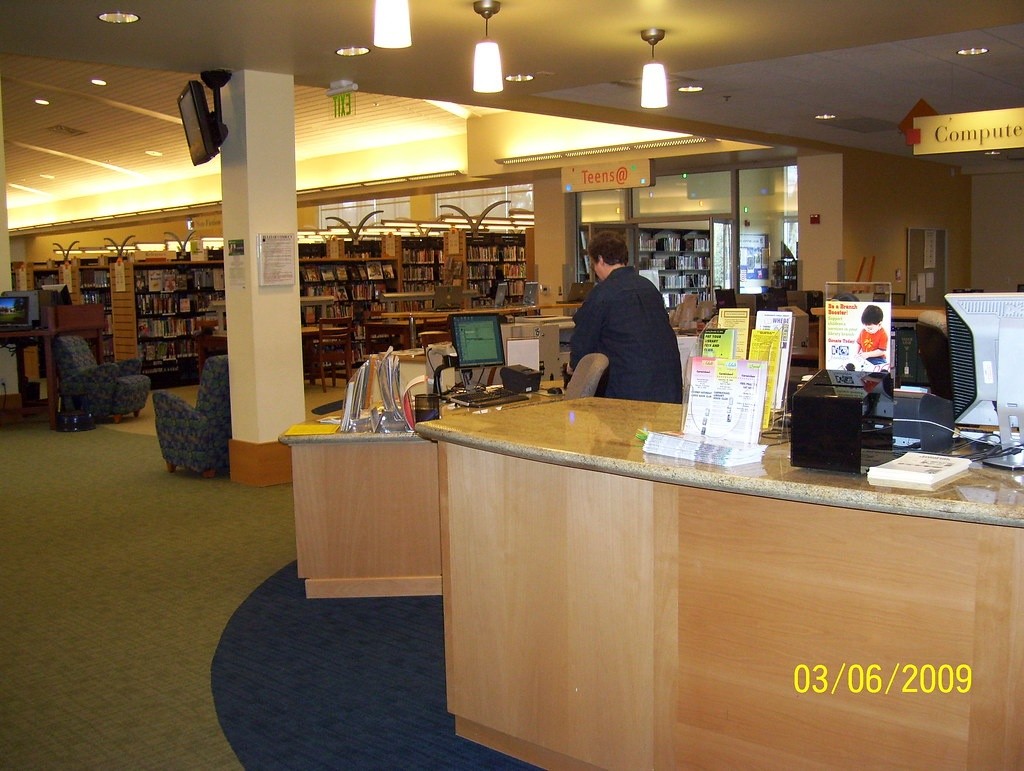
[
  {"x1": 638, "y1": 235, "x2": 711, "y2": 307},
  {"x1": 300, "y1": 246, "x2": 526, "y2": 367},
  {"x1": 341, "y1": 382, "x2": 355, "y2": 432},
  {"x1": 34, "y1": 268, "x2": 225, "y2": 373}
]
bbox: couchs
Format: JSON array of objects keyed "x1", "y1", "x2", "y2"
[
  {"x1": 52, "y1": 333, "x2": 150, "y2": 423},
  {"x1": 152, "y1": 353, "x2": 230, "y2": 478}
]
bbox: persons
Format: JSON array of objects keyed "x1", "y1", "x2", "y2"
[
  {"x1": 857, "y1": 305, "x2": 887, "y2": 365},
  {"x1": 566, "y1": 232, "x2": 682, "y2": 404}
]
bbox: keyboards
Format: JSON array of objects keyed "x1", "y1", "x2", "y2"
[
  {"x1": 952, "y1": 429, "x2": 1021, "y2": 445},
  {"x1": 450, "y1": 388, "x2": 529, "y2": 408}
]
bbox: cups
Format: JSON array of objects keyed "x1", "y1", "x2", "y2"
[{"x1": 414, "y1": 394, "x2": 439, "y2": 422}]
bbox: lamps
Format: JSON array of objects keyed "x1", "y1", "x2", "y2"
[
  {"x1": 641, "y1": 28, "x2": 667, "y2": 108},
  {"x1": 472, "y1": 0, "x2": 503, "y2": 92},
  {"x1": 374, "y1": 0, "x2": 412, "y2": 47}
]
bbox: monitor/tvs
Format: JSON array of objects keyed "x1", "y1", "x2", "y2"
[
  {"x1": 449, "y1": 313, "x2": 506, "y2": 371},
  {"x1": 762, "y1": 286, "x2": 788, "y2": 311},
  {"x1": 0, "y1": 289, "x2": 40, "y2": 330},
  {"x1": 714, "y1": 288, "x2": 737, "y2": 312},
  {"x1": 177, "y1": 79, "x2": 221, "y2": 167},
  {"x1": 945, "y1": 292, "x2": 1024, "y2": 471},
  {"x1": 42, "y1": 284, "x2": 72, "y2": 305}
]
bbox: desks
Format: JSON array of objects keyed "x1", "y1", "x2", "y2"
[
  {"x1": 0, "y1": 304, "x2": 112, "y2": 428},
  {"x1": 280, "y1": 404, "x2": 443, "y2": 600},
  {"x1": 381, "y1": 307, "x2": 532, "y2": 348},
  {"x1": 196, "y1": 325, "x2": 348, "y2": 385},
  {"x1": 538, "y1": 304, "x2": 583, "y2": 316},
  {"x1": 362, "y1": 318, "x2": 449, "y2": 353}
]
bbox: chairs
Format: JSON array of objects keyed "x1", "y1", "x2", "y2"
[{"x1": 308, "y1": 314, "x2": 353, "y2": 392}]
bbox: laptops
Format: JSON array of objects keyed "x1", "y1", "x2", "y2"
[
  {"x1": 510, "y1": 281, "x2": 539, "y2": 306},
  {"x1": 477, "y1": 284, "x2": 510, "y2": 309},
  {"x1": 423, "y1": 286, "x2": 464, "y2": 312}
]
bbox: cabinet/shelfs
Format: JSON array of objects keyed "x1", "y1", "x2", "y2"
[{"x1": 13, "y1": 230, "x2": 711, "y2": 387}]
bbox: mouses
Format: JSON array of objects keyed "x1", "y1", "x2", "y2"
[{"x1": 548, "y1": 387, "x2": 562, "y2": 394}]
